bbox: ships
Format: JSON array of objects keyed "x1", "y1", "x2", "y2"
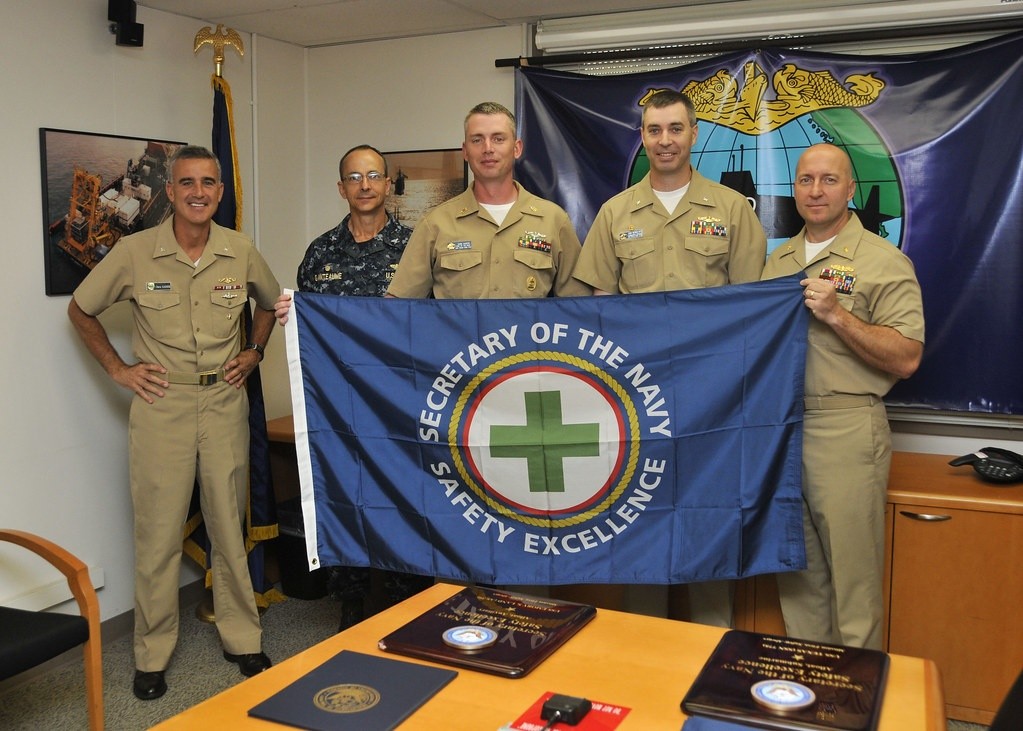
[{"x1": 48, "y1": 141, "x2": 179, "y2": 277}]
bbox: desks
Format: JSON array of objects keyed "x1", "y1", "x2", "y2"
[{"x1": 144, "y1": 582, "x2": 945, "y2": 731}]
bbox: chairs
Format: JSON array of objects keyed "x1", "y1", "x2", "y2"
[{"x1": 0, "y1": 528, "x2": 105, "y2": 731}]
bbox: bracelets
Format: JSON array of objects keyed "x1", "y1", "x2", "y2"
[{"x1": 244, "y1": 343, "x2": 265, "y2": 362}]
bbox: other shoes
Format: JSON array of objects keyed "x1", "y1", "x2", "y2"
[{"x1": 338, "y1": 593, "x2": 363, "y2": 632}]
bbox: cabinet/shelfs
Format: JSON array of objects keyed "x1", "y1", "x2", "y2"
[{"x1": 579, "y1": 450, "x2": 1023, "y2": 725}]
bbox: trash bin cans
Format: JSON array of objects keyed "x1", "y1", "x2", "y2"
[{"x1": 274, "y1": 495, "x2": 329, "y2": 601}]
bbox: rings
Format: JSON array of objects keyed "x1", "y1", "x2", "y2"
[{"x1": 810, "y1": 291, "x2": 816, "y2": 299}]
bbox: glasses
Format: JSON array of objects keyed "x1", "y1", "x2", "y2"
[{"x1": 340, "y1": 170, "x2": 385, "y2": 184}]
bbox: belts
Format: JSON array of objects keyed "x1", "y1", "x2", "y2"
[
  {"x1": 147, "y1": 367, "x2": 238, "y2": 386},
  {"x1": 803, "y1": 392, "x2": 883, "y2": 411}
]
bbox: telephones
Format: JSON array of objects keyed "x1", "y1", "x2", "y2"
[{"x1": 947, "y1": 447, "x2": 1023, "y2": 485}]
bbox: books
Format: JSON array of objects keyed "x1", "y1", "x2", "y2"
[
  {"x1": 679, "y1": 629, "x2": 890, "y2": 731},
  {"x1": 378, "y1": 586, "x2": 598, "y2": 678},
  {"x1": 248, "y1": 651, "x2": 458, "y2": 731}
]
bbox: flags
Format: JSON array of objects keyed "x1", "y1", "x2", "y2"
[
  {"x1": 175, "y1": 79, "x2": 289, "y2": 610},
  {"x1": 282, "y1": 289, "x2": 808, "y2": 586}
]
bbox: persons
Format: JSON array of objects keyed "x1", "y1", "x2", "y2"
[
  {"x1": 570, "y1": 87, "x2": 767, "y2": 632},
  {"x1": 67, "y1": 144, "x2": 281, "y2": 695},
  {"x1": 759, "y1": 142, "x2": 926, "y2": 651},
  {"x1": 274, "y1": 145, "x2": 412, "y2": 633},
  {"x1": 385, "y1": 101, "x2": 581, "y2": 599}
]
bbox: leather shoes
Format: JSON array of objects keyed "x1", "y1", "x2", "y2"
[
  {"x1": 133, "y1": 668, "x2": 168, "y2": 700},
  {"x1": 223, "y1": 647, "x2": 272, "y2": 678}
]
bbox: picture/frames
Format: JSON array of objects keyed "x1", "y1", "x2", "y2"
[
  {"x1": 39, "y1": 127, "x2": 188, "y2": 297},
  {"x1": 381, "y1": 148, "x2": 469, "y2": 231}
]
